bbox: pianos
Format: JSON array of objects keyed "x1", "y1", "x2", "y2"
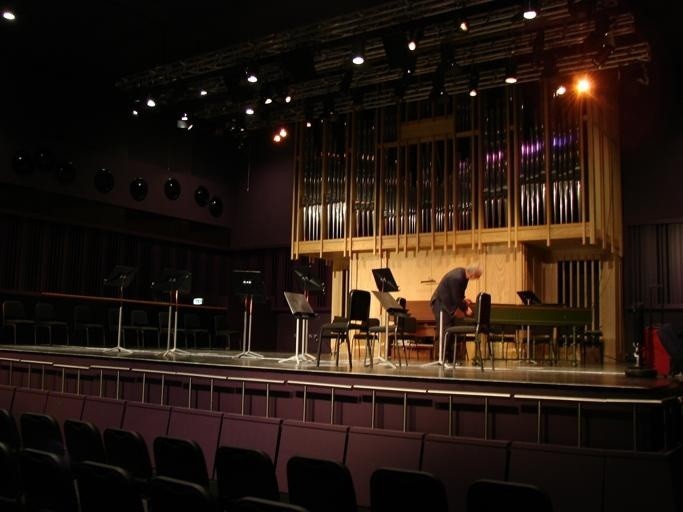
[{"x1": 380, "y1": 301, "x2": 465, "y2": 362}]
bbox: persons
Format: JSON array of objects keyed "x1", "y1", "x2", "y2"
[{"x1": 430, "y1": 260, "x2": 483, "y2": 363}]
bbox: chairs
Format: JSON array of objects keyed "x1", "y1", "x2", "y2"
[
  {"x1": 369, "y1": 467, "x2": 449, "y2": 512},
  {"x1": 80, "y1": 462, "x2": 144, "y2": 512},
  {"x1": 148, "y1": 475, "x2": 210, "y2": 512},
  {"x1": 465, "y1": 478, "x2": 547, "y2": 512},
  {"x1": 3, "y1": 443, "x2": 22, "y2": 512},
  {"x1": 102, "y1": 427, "x2": 152, "y2": 499},
  {"x1": 1, "y1": 408, "x2": 20, "y2": 450},
  {"x1": 65, "y1": 420, "x2": 104, "y2": 478},
  {"x1": 285, "y1": 454, "x2": 359, "y2": 512},
  {"x1": 18, "y1": 448, "x2": 78, "y2": 512},
  {"x1": 151, "y1": 437, "x2": 214, "y2": 512},
  {"x1": 315, "y1": 289, "x2": 606, "y2": 368},
  {"x1": 239, "y1": 496, "x2": 306, "y2": 512},
  {"x1": 1, "y1": 299, "x2": 244, "y2": 352},
  {"x1": 19, "y1": 409, "x2": 71, "y2": 467},
  {"x1": 215, "y1": 444, "x2": 282, "y2": 511}
]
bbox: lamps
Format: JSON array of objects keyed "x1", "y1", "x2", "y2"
[{"x1": 125, "y1": 1, "x2": 615, "y2": 133}]
